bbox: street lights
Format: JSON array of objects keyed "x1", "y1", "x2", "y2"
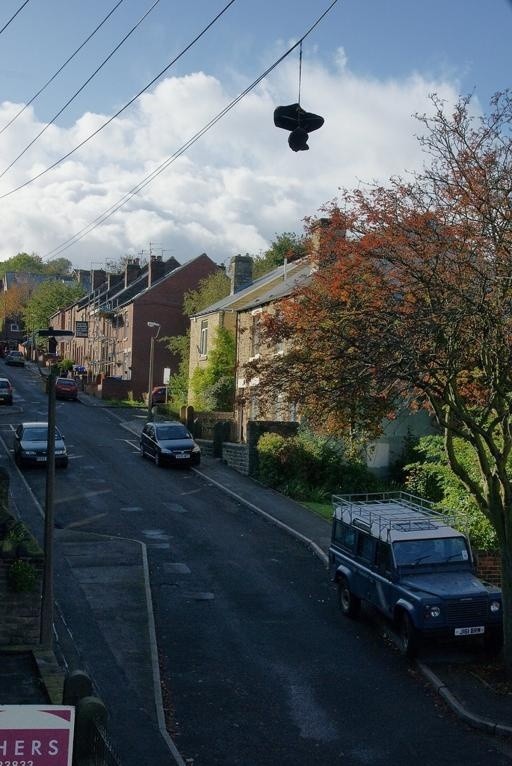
[
  {"x1": 147, "y1": 322, "x2": 160, "y2": 419},
  {"x1": 37, "y1": 324, "x2": 74, "y2": 642}
]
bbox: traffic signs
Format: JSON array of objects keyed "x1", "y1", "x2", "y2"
[{"x1": 76, "y1": 321, "x2": 88, "y2": 338}]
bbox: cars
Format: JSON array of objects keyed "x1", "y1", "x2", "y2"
[
  {"x1": 14, "y1": 422, "x2": 68, "y2": 468},
  {"x1": 56, "y1": 377, "x2": 77, "y2": 401},
  {"x1": 0, "y1": 378, "x2": 13, "y2": 406},
  {"x1": 141, "y1": 421, "x2": 201, "y2": 468},
  {"x1": 6, "y1": 350, "x2": 25, "y2": 368}
]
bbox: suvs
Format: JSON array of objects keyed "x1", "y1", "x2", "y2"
[{"x1": 329, "y1": 491, "x2": 504, "y2": 657}]
